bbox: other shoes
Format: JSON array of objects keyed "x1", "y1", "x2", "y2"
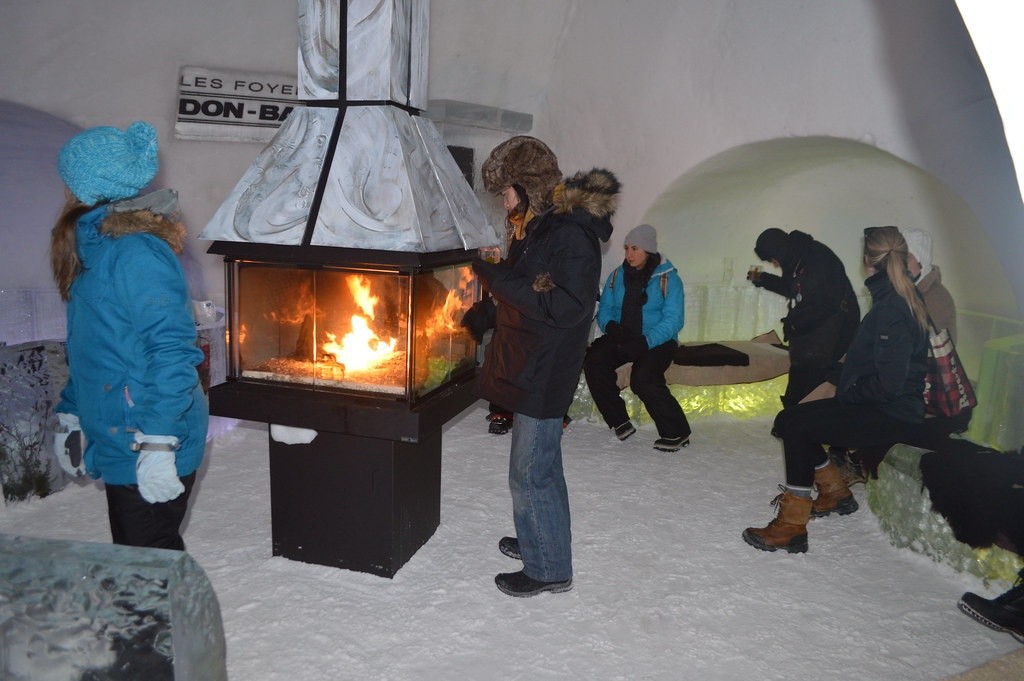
[
  {"x1": 563, "y1": 415, "x2": 573, "y2": 428},
  {"x1": 614, "y1": 420, "x2": 636, "y2": 441},
  {"x1": 486, "y1": 412, "x2": 513, "y2": 434},
  {"x1": 79, "y1": 641, "x2": 175, "y2": 681},
  {"x1": 653, "y1": 430, "x2": 691, "y2": 452}
]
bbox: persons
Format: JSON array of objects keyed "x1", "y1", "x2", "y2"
[
  {"x1": 741, "y1": 225, "x2": 929, "y2": 554},
  {"x1": 825, "y1": 225, "x2": 956, "y2": 486},
  {"x1": 956, "y1": 566, "x2": 1024, "y2": 646},
  {"x1": 583, "y1": 224, "x2": 692, "y2": 452},
  {"x1": 47, "y1": 120, "x2": 209, "y2": 681},
  {"x1": 460, "y1": 136, "x2": 622, "y2": 598},
  {"x1": 747, "y1": 228, "x2": 860, "y2": 409}
]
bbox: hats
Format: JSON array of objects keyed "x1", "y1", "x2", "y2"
[
  {"x1": 481, "y1": 135, "x2": 562, "y2": 216},
  {"x1": 754, "y1": 227, "x2": 788, "y2": 261},
  {"x1": 623, "y1": 223, "x2": 657, "y2": 253},
  {"x1": 55, "y1": 120, "x2": 160, "y2": 207}
]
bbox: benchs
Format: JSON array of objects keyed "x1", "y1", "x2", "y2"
[
  {"x1": 868, "y1": 431, "x2": 1024, "y2": 582},
  {"x1": 570, "y1": 330, "x2": 791, "y2": 424}
]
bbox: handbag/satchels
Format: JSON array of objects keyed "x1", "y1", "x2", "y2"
[{"x1": 922, "y1": 327, "x2": 978, "y2": 417}]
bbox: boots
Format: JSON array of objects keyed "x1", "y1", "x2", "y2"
[
  {"x1": 742, "y1": 484, "x2": 814, "y2": 553},
  {"x1": 811, "y1": 460, "x2": 859, "y2": 517}
]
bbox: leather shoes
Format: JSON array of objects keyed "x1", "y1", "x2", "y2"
[{"x1": 958, "y1": 574, "x2": 1024, "y2": 643}]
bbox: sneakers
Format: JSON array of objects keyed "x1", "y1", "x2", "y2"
[
  {"x1": 499, "y1": 536, "x2": 524, "y2": 560},
  {"x1": 494, "y1": 568, "x2": 573, "y2": 598}
]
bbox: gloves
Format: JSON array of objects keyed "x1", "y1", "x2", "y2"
[
  {"x1": 54, "y1": 412, "x2": 86, "y2": 478},
  {"x1": 616, "y1": 334, "x2": 648, "y2": 361},
  {"x1": 472, "y1": 256, "x2": 512, "y2": 275},
  {"x1": 460, "y1": 299, "x2": 497, "y2": 345},
  {"x1": 746, "y1": 270, "x2": 762, "y2": 288},
  {"x1": 130, "y1": 431, "x2": 185, "y2": 504},
  {"x1": 604, "y1": 320, "x2": 627, "y2": 342},
  {"x1": 781, "y1": 316, "x2": 799, "y2": 342}
]
